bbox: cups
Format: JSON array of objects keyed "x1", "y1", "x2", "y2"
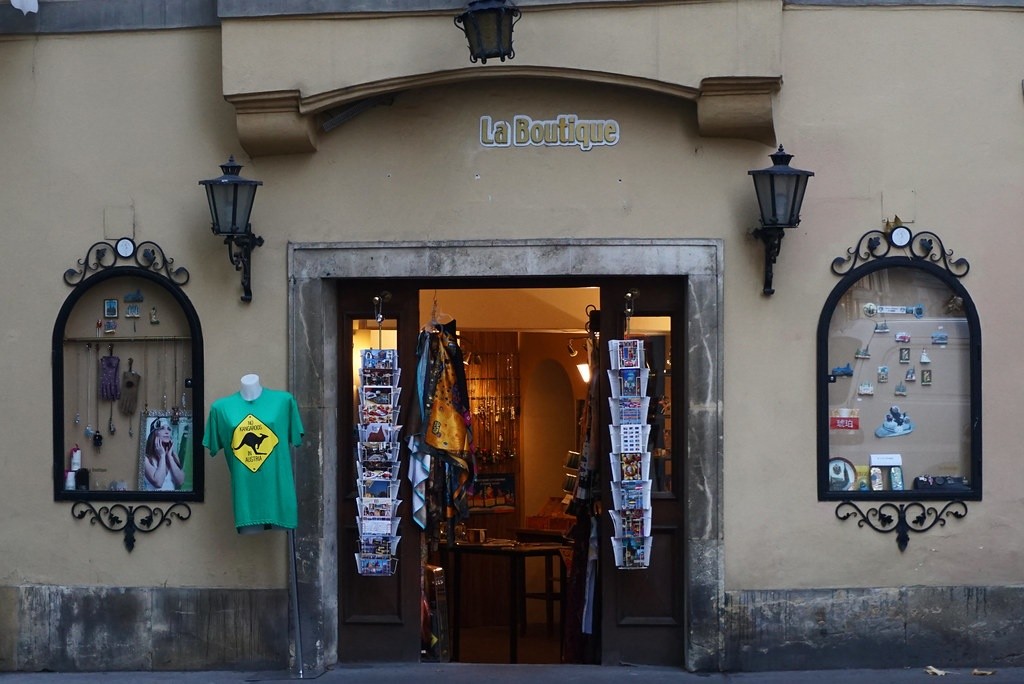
[{"x1": 64, "y1": 449, "x2": 89, "y2": 491}]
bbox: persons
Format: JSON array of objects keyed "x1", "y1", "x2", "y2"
[
  {"x1": 203, "y1": 374, "x2": 305, "y2": 533},
  {"x1": 144, "y1": 419, "x2": 185, "y2": 492}
]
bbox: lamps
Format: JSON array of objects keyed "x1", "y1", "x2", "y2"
[
  {"x1": 451, "y1": 0, "x2": 523, "y2": 65},
  {"x1": 746, "y1": 141, "x2": 815, "y2": 297},
  {"x1": 198, "y1": 152, "x2": 265, "y2": 304},
  {"x1": 568, "y1": 338, "x2": 579, "y2": 357},
  {"x1": 462, "y1": 350, "x2": 483, "y2": 367}
]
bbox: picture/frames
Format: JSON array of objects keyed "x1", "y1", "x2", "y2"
[
  {"x1": 104, "y1": 298, "x2": 118, "y2": 318},
  {"x1": 137, "y1": 409, "x2": 193, "y2": 492},
  {"x1": 829, "y1": 456, "x2": 856, "y2": 491},
  {"x1": 899, "y1": 347, "x2": 912, "y2": 364},
  {"x1": 921, "y1": 369, "x2": 933, "y2": 386},
  {"x1": 870, "y1": 466, "x2": 884, "y2": 490},
  {"x1": 889, "y1": 466, "x2": 905, "y2": 490}
]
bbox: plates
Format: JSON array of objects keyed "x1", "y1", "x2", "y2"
[{"x1": 828, "y1": 457, "x2": 857, "y2": 491}]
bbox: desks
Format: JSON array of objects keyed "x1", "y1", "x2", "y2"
[
  {"x1": 436, "y1": 540, "x2": 569, "y2": 664},
  {"x1": 507, "y1": 527, "x2": 568, "y2": 640}
]
